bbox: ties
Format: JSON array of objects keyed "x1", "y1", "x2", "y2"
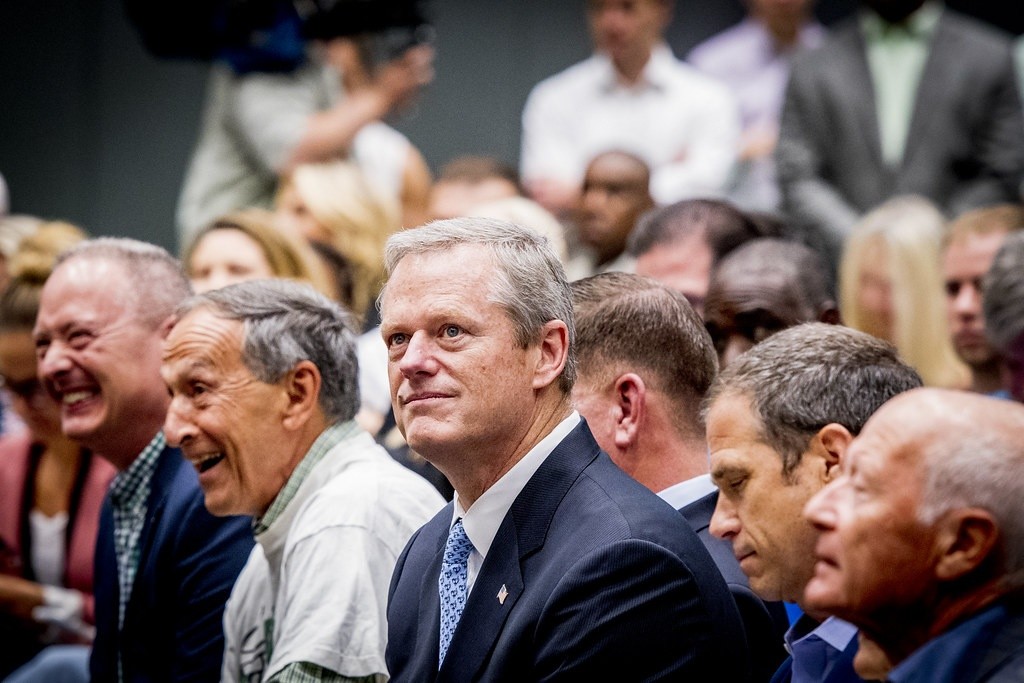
[{"x1": 437, "y1": 521, "x2": 476, "y2": 671}]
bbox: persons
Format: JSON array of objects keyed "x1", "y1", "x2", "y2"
[
  {"x1": 1, "y1": 215, "x2": 122, "y2": 683},
  {"x1": 563, "y1": 270, "x2": 791, "y2": 683},
  {"x1": 32, "y1": 237, "x2": 255, "y2": 683},
  {"x1": 380, "y1": 213, "x2": 737, "y2": 683},
  {"x1": 802, "y1": 387, "x2": 1023, "y2": 683},
  {"x1": 176, "y1": 0, "x2": 1023, "y2": 503},
  {"x1": 160, "y1": 279, "x2": 449, "y2": 683},
  {"x1": 700, "y1": 322, "x2": 923, "y2": 683}
]
